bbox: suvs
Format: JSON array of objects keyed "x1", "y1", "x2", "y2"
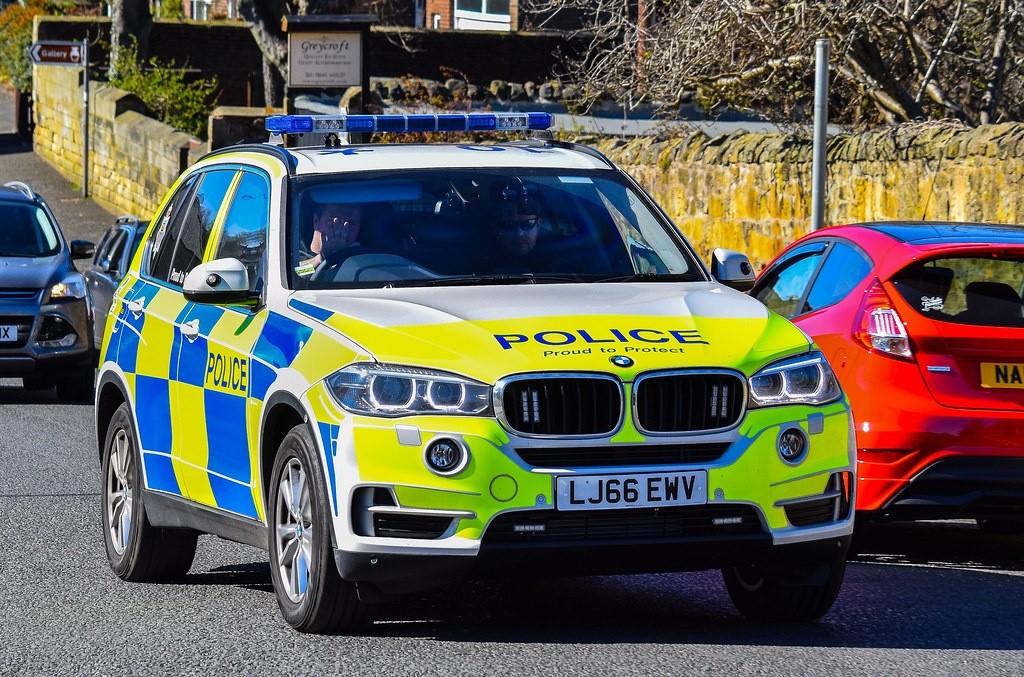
[
  {"x1": 83, "y1": 215, "x2": 151, "y2": 351},
  {"x1": 0, "y1": 181, "x2": 97, "y2": 402}
]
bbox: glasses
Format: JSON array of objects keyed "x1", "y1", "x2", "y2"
[{"x1": 498, "y1": 216, "x2": 539, "y2": 231}]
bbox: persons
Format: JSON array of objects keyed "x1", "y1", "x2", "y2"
[
  {"x1": 299, "y1": 201, "x2": 364, "y2": 279},
  {"x1": 474, "y1": 197, "x2": 584, "y2": 274}
]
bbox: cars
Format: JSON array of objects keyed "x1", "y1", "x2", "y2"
[
  {"x1": 743, "y1": 220, "x2": 1024, "y2": 533},
  {"x1": 92, "y1": 112, "x2": 856, "y2": 635}
]
